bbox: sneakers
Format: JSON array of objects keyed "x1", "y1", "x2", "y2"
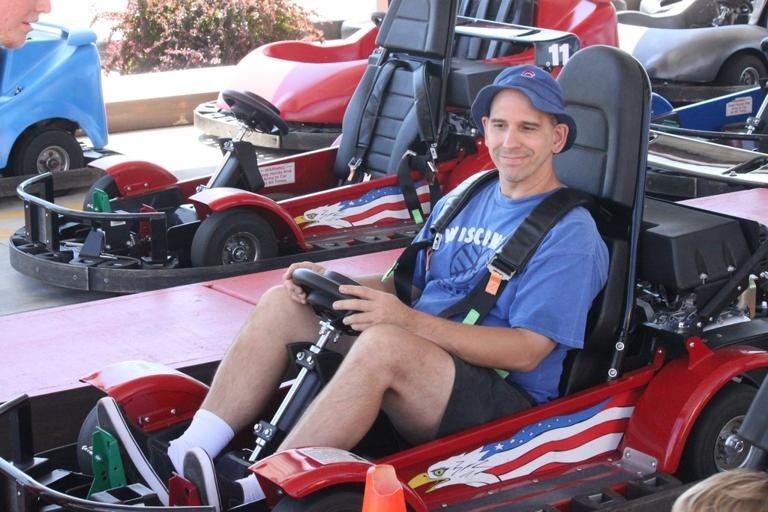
[
  {"x1": 180, "y1": 445, "x2": 245, "y2": 512},
  {"x1": 95, "y1": 395, "x2": 180, "y2": 508}
]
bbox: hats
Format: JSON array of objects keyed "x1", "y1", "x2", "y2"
[{"x1": 470, "y1": 63, "x2": 577, "y2": 154}]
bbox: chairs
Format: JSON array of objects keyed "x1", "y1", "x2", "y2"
[
  {"x1": 325, "y1": 1, "x2": 458, "y2": 187},
  {"x1": 734, "y1": 370, "x2": 768, "y2": 476},
  {"x1": 534, "y1": 42, "x2": 655, "y2": 398}
]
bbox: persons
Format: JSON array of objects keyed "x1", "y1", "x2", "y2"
[
  {"x1": 0, "y1": 0, "x2": 51, "y2": 48},
  {"x1": 97, "y1": 65, "x2": 610, "y2": 511},
  {"x1": 671, "y1": 468, "x2": 768, "y2": 512}
]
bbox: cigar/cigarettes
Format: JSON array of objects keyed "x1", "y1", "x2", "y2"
[{"x1": 29, "y1": 22, "x2": 61, "y2": 34}]
